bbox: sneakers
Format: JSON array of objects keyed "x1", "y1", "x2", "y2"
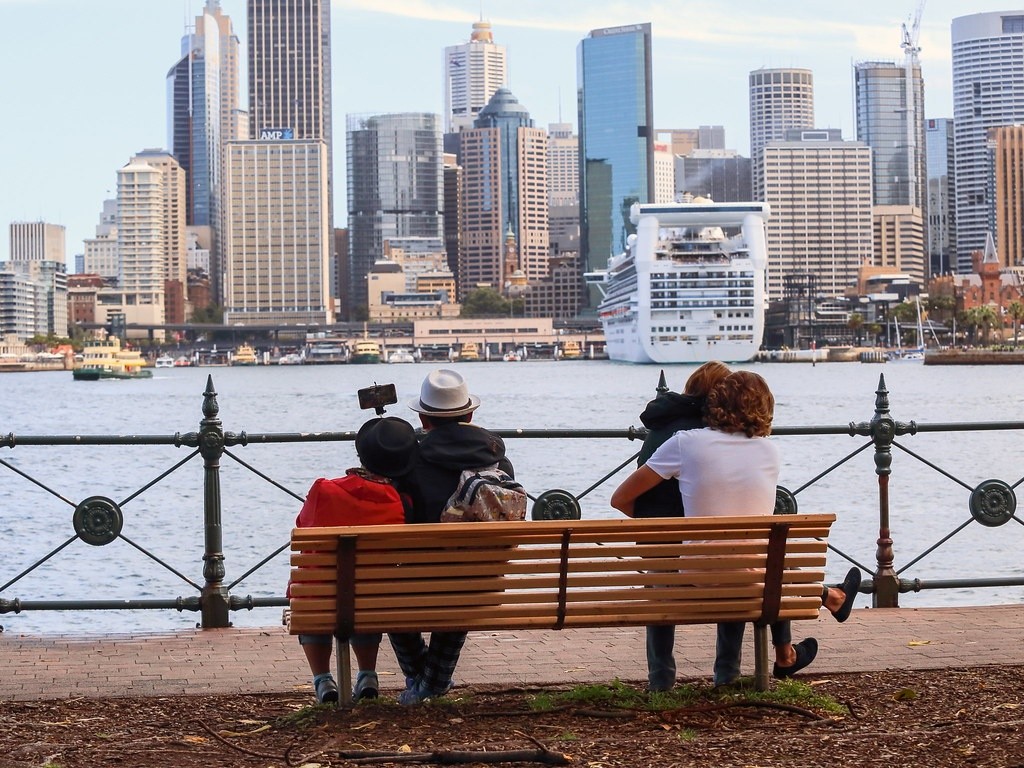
[
  {"x1": 830, "y1": 566, "x2": 861, "y2": 623},
  {"x1": 772, "y1": 637, "x2": 819, "y2": 680}
]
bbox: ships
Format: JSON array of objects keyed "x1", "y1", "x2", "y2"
[{"x1": 582, "y1": 199, "x2": 773, "y2": 364}]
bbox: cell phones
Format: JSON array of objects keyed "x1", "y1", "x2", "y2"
[{"x1": 358, "y1": 384, "x2": 397, "y2": 410}]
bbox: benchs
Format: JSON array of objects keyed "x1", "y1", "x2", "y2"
[{"x1": 282, "y1": 513, "x2": 836, "y2": 712}]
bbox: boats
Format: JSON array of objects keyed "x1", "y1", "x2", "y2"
[
  {"x1": 73, "y1": 368, "x2": 99, "y2": 381},
  {"x1": 561, "y1": 341, "x2": 581, "y2": 361},
  {"x1": 155, "y1": 354, "x2": 191, "y2": 368},
  {"x1": 503, "y1": 350, "x2": 521, "y2": 362},
  {"x1": 351, "y1": 321, "x2": 380, "y2": 363},
  {"x1": 73, "y1": 327, "x2": 154, "y2": 379},
  {"x1": 461, "y1": 342, "x2": 478, "y2": 362},
  {"x1": 232, "y1": 342, "x2": 258, "y2": 366},
  {"x1": 279, "y1": 354, "x2": 302, "y2": 366},
  {"x1": 390, "y1": 348, "x2": 414, "y2": 363}
]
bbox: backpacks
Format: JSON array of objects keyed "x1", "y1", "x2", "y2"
[{"x1": 440, "y1": 462, "x2": 527, "y2": 577}]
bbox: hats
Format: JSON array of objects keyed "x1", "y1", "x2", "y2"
[
  {"x1": 408, "y1": 368, "x2": 481, "y2": 416},
  {"x1": 354, "y1": 416, "x2": 419, "y2": 476}
]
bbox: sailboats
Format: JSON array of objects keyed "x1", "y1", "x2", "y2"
[{"x1": 884, "y1": 294, "x2": 941, "y2": 361}]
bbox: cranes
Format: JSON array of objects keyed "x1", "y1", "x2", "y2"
[{"x1": 900, "y1": 0, "x2": 928, "y2": 64}]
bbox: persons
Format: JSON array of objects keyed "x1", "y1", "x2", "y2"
[
  {"x1": 391, "y1": 369, "x2": 515, "y2": 704},
  {"x1": 610, "y1": 371, "x2": 779, "y2": 692},
  {"x1": 633, "y1": 359, "x2": 861, "y2": 679},
  {"x1": 296, "y1": 417, "x2": 417, "y2": 702}
]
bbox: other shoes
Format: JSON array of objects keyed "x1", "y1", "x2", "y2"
[
  {"x1": 400, "y1": 675, "x2": 455, "y2": 704},
  {"x1": 717, "y1": 670, "x2": 755, "y2": 693},
  {"x1": 314, "y1": 676, "x2": 338, "y2": 703},
  {"x1": 354, "y1": 675, "x2": 378, "y2": 699},
  {"x1": 641, "y1": 686, "x2": 674, "y2": 705}
]
